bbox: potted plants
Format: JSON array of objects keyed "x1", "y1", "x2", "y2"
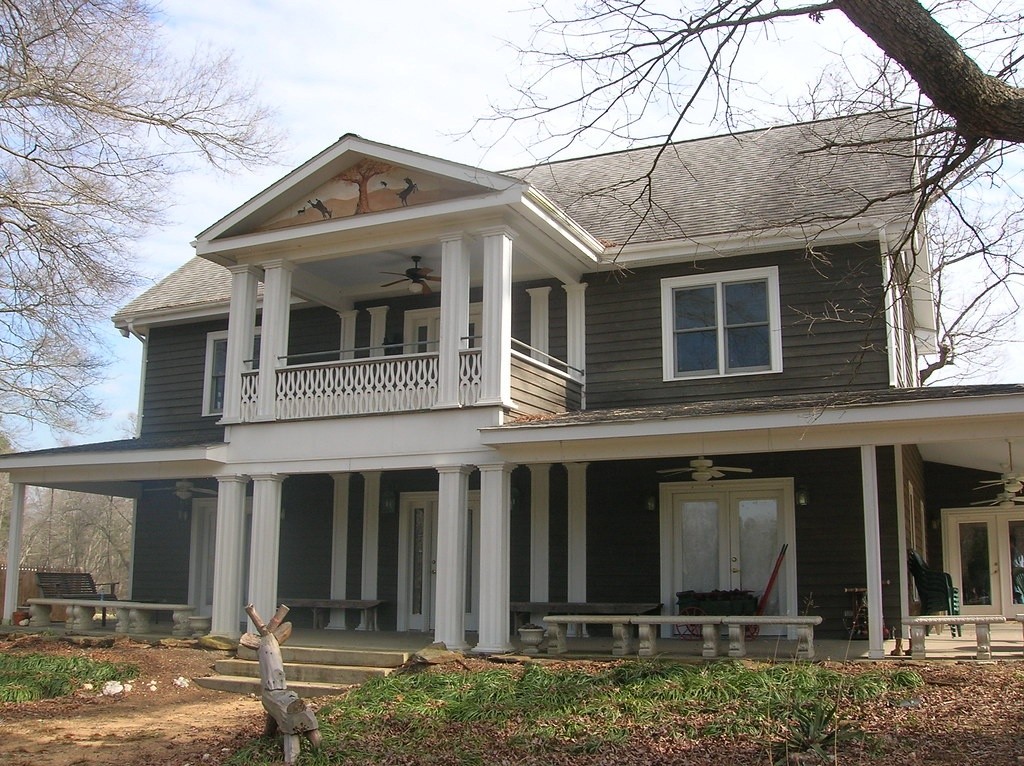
[{"x1": 518, "y1": 623, "x2": 547, "y2": 647}]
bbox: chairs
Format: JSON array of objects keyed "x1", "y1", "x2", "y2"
[{"x1": 907, "y1": 548, "x2": 963, "y2": 638}]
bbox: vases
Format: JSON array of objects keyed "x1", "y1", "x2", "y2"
[
  {"x1": 13, "y1": 612, "x2": 29, "y2": 626},
  {"x1": 188, "y1": 616, "x2": 212, "y2": 637}
]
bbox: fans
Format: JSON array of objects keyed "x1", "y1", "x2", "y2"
[
  {"x1": 970, "y1": 493, "x2": 1024, "y2": 506},
  {"x1": 144, "y1": 480, "x2": 217, "y2": 495},
  {"x1": 657, "y1": 457, "x2": 752, "y2": 478},
  {"x1": 972, "y1": 471, "x2": 1024, "y2": 490},
  {"x1": 379, "y1": 256, "x2": 441, "y2": 293}
]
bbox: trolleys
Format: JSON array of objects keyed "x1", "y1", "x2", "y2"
[{"x1": 671, "y1": 543, "x2": 790, "y2": 642}]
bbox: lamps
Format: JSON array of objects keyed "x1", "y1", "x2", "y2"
[
  {"x1": 408, "y1": 283, "x2": 423, "y2": 292},
  {"x1": 119, "y1": 329, "x2": 130, "y2": 338},
  {"x1": 692, "y1": 472, "x2": 712, "y2": 481},
  {"x1": 1000, "y1": 502, "x2": 1015, "y2": 509},
  {"x1": 646, "y1": 493, "x2": 655, "y2": 510},
  {"x1": 1004, "y1": 484, "x2": 1023, "y2": 492},
  {"x1": 383, "y1": 334, "x2": 402, "y2": 355},
  {"x1": 175, "y1": 491, "x2": 192, "y2": 499},
  {"x1": 796, "y1": 484, "x2": 810, "y2": 506}
]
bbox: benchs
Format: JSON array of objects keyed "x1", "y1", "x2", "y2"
[
  {"x1": 1015, "y1": 614, "x2": 1024, "y2": 640},
  {"x1": 35, "y1": 572, "x2": 120, "y2": 627},
  {"x1": 901, "y1": 615, "x2": 1006, "y2": 659},
  {"x1": 277, "y1": 598, "x2": 387, "y2": 632},
  {"x1": 510, "y1": 602, "x2": 663, "y2": 639},
  {"x1": 26, "y1": 598, "x2": 196, "y2": 637},
  {"x1": 543, "y1": 615, "x2": 822, "y2": 659}
]
